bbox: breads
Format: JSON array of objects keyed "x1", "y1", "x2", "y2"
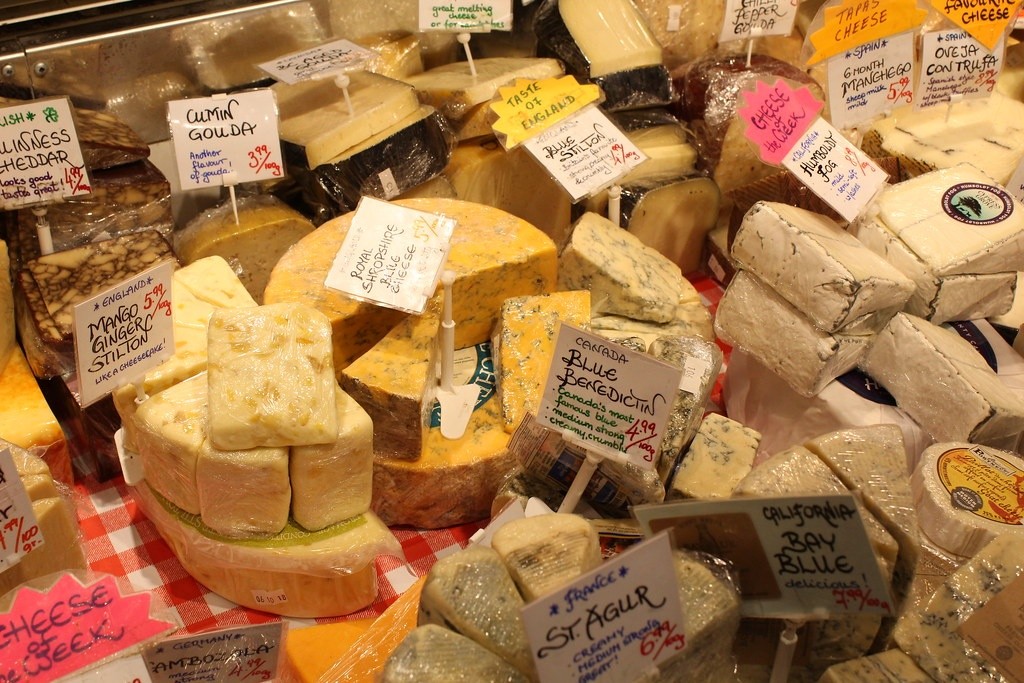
[{"x1": 0, "y1": 0, "x2": 1024, "y2": 683}]
